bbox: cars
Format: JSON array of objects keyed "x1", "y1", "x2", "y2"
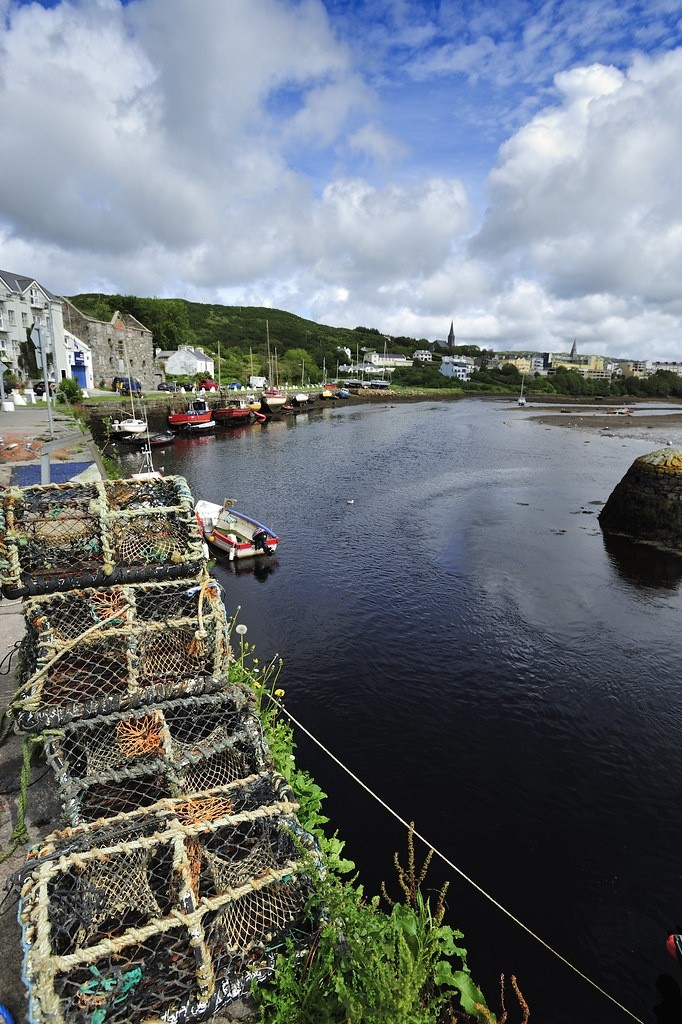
[
  {"x1": 177, "y1": 382, "x2": 193, "y2": 392},
  {"x1": 228, "y1": 381, "x2": 241, "y2": 390},
  {"x1": 158, "y1": 383, "x2": 176, "y2": 390},
  {"x1": 34, "y1": 382, "x2": 59, "y2": 396}
]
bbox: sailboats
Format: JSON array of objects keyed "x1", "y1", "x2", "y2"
[
  {"x1": 346, "y1": 344, "x2": 368, "y2": 388},
  {"x1": 293, "y1": 357, "x2": 309, "y2": 404},
  {"x1": 370, "y1": 341, "x2": 392, "y2": 390},
  {"x1": 322, "y1": 358, "x2": 331, "y2": 398},
  {"x1": 110, "y1": 339, "x2": 148, "y2": 434},
  {"x1": 212, "y1": 340, "x2": 251, "y2": 422},
  {"x1": 259, "y1": 319, "x2": 287, "y2": 413},
  {"x1": 517, "y1": 373, "x2": 526, "y2": 405}
]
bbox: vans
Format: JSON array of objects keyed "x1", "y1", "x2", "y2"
[
  {"x1": 200, "y1": 379, "x2": 218, "y2": 390},
  {"x1": 112, "y1": 377, "x2": 142, "y2": 395}
]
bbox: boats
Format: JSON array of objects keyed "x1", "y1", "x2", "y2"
[
  {"x1": 338, "y1": 387, "x2": 349, "y2": 398},
  {"x1": 122, "y1": 430, "x2": 161, "y2": 441},
  {"x1": 194, "y1": 500, "x2": 280, "y2": 561},
  {"x1": 146, "y1": 434, "x2": 175, "y2": 445},
  {"x1": 168, "y1": 393, "x2": 212, "y2": 426},
  {"x1": 608, "y1": 406, "x2": 631, "y2": 414}
]
